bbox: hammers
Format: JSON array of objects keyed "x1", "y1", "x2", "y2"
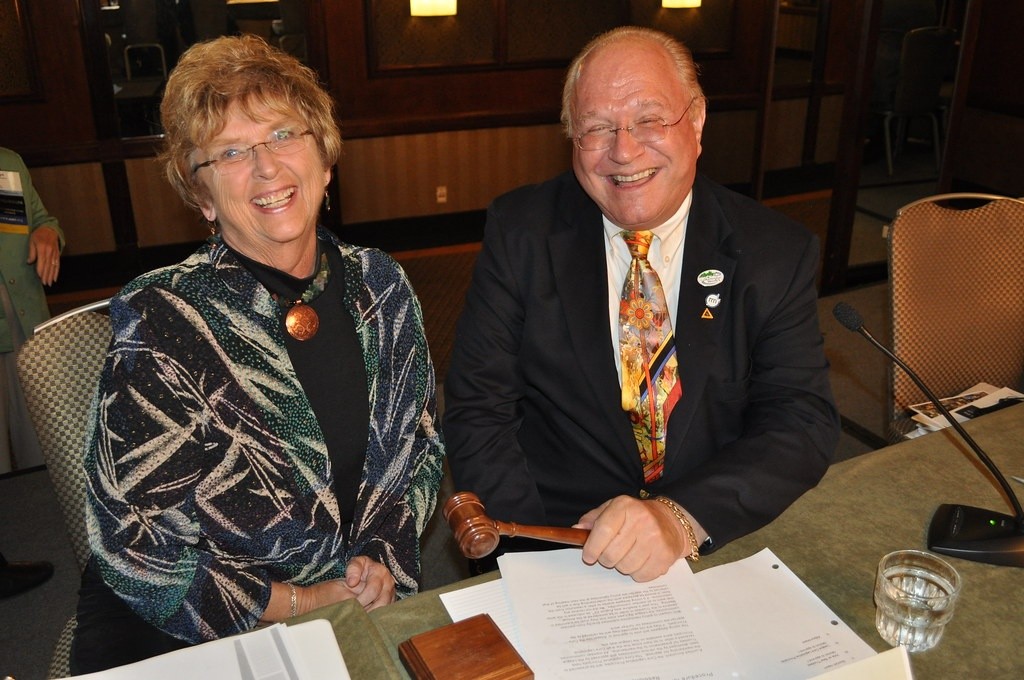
[{"x1": 442, "y1": 492, "x2": 591, "y2": 560}]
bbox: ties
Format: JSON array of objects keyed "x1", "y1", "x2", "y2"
[{"x1": 618, "y1": 229, "x2": 683, "y2": 486}]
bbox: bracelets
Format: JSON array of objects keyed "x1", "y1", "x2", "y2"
[
  {"x1": 287, "y1": 582, "x2": 296, "y2": 617},
  {"x1": 650, "y1": 495, "x2": 700, "y2": 563}
]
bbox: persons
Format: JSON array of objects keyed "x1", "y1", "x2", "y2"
[
  {"x1": 443, "y1": 25, "x2": 841, "y2": 583},
  {"x1": 68, "y1": 33, "x2": 447, "y2": 677},
  {"x1": 0, "y1": 146, "x2": 66, "y2": 600}
]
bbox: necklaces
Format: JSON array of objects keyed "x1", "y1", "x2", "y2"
[{"x1": 273, "y1": 251, "x2": 331, "y2": 341}]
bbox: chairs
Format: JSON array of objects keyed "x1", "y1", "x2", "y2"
[
  {"x1": 867, "y1": 24, "x2": 958, "y2": 180},
  {"x1": 124, "y1": 43, "x2": 167, "y2": 135},
  {"x1": 882, "y1": 193, "x2": 1024, "y2": 446},
  {"x1": 15, "y1": 297, "x2": 112, "y2": 679}
]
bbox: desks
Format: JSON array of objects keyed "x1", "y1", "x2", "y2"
[
  {"x1": 114, "y1": 79, "x2": 163, "y2": 138},
  {"x1": 370, "y1": 402, "x2": 1024, "y2": 680}
]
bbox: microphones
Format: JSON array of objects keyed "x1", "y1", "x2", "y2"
[{"x1": 834, "y1": 301, "x2": 1024, "y2": 568}]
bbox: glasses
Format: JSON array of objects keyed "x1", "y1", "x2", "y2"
[
  {"x1": 572, "y1": 94, "x2": 696, "y2": 151},
  {"x1": 192, "y1": 124, "x2": 314, "y2": 176}
]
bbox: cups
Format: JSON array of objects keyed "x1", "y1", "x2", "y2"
[{"x1": 873, "y1": 549, "x2": 961, "y2": 653}]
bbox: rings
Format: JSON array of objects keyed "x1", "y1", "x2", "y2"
[{"x1": 53, "y1": 262, "x2": 56, "y2": 266}]
